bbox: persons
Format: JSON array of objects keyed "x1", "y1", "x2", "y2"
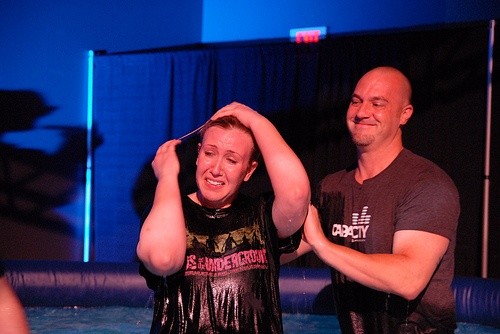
[
  {"x1": 137, "y1": 103, "x2": 310, "y2": 334},
  {"x1": 280, "y1": 67, "x2": 460, "y2": 334}
]
